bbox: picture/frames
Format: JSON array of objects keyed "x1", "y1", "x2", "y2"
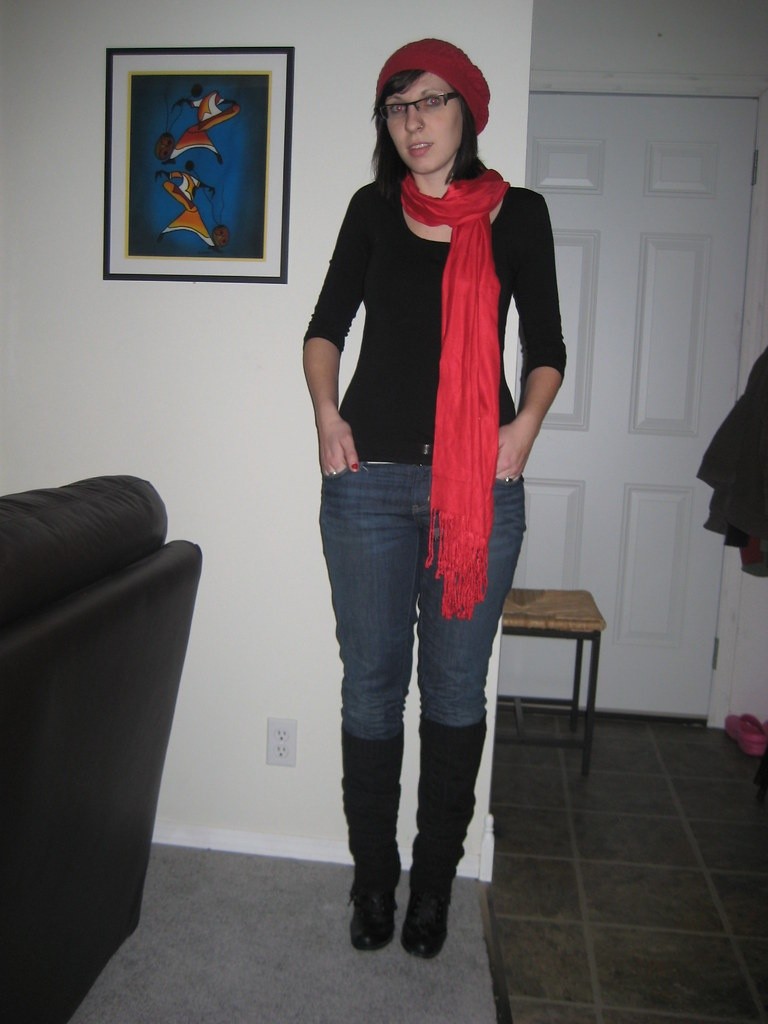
[{"x1": 102, "y1": 48, "x2": 297, "y2": 285}]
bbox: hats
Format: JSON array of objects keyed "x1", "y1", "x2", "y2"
[{"x1": 376, "y1": 38, "x2": 491, "y2": 136}]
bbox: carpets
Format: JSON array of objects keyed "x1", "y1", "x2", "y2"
[{"x1": 69, "y1": 842, "x2": 515, "y2": 1024}]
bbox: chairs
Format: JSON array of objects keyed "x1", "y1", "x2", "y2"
[{"x1": 494, "y1": 588, "x2": 607, "y2": 775}]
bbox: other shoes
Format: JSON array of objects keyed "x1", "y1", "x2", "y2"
[{"x1": 726, "y1": 714, "x2": 768, "y2": 757}]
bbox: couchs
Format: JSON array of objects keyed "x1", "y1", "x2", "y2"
[{"x1": 0, "y1": 475, "x2": 202, "y2": 1024}]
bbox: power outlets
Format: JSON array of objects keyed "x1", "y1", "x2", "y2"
[{"x1": 265, "y1": 718, "x2": 297, "y2": 766}]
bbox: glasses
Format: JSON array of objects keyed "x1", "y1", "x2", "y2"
[{"x1": 380, "y1": 92, "x2": 460, "y2": 120}]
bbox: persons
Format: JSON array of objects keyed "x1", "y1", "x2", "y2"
[{"x1": 302, "y1": 39, "x2": 566, "y2": 956}]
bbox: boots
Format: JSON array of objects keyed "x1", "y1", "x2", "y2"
[
  {"x1": 341, "y1": 723, "x2": 405, "y2": 952},
  {"x1": 401, "y1": 714, "x2": 488, "y2": 958}
]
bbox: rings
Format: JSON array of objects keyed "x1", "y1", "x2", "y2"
[
  {"x1": 329, "y1": 472, "x2": 336, "y2": 476},
  {"x1": 505, "y1": 478, "x2": 512, "y2": 482}
]
